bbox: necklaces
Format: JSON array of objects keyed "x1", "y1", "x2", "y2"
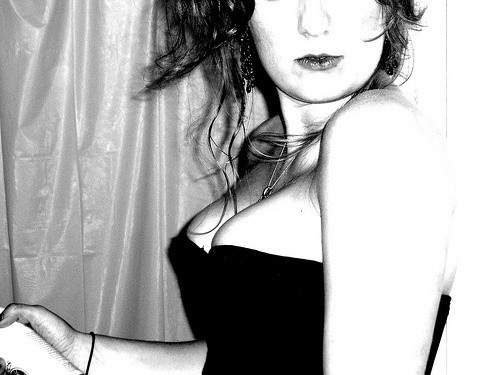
[{"x1": 262, "y1": 124, "x2": 325, "y2": 202}]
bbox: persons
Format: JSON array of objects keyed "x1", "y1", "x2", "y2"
[{"x1": 0, "y1": 0, "x2": 453, "y2": 375}]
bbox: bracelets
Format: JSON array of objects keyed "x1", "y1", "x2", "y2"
[{"x1": 83, "y1": 331, "x2": 97, "y2": 375}]
